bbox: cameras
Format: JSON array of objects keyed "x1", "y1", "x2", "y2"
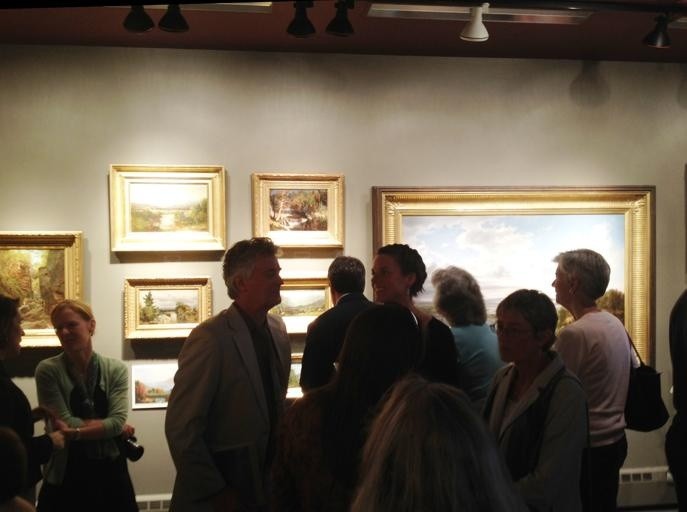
[{"x1": 113, "y1": 435, "x2": 144, "y2": 462}]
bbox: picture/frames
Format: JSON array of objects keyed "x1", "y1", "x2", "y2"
[
  {"x1": 267, "y1": 278, "x2": 336, "y2": 336},
  {"x1": 132, "y1": 359, "x2": 180, "y2": 412},
  {"x1": 108, "y1": 161, "x2": 227, "y2": 254},
  {"x1": 250, "y1": 172, "x2": 346, "y2": 251},
  {"x1": 0, "y1": 231, "x2": 84, "y2": 353},
  {"x1": 371, "y1": 186, "x2": 655, "y2": 367},
  {"x1": 281, "y1": 352, "x2": 310, "y2": 403},
  {"x1": 122, "y1": 276, "x2": 214, "y2": 341}
]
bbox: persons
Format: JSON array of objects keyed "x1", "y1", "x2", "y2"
[
  {"x1": 0, "y1": 427, "x2": 35, "y2": 512},
  {"x1": 0, "y1": 295, "x2": 64, "y2": 486},
  {"x1": 278, "y1": 303, "x2": 422, "y2": 509},
  {"x1": 298, "y1": 256, "x2": 371, "y2": 391},
  {"x1": 163, "y1": 238, "x2": 292, "y2": 512},
  {"x1": 552, "y1": 248, "x2": 639, "y2": 512},
  {"x1": 664, "y1": 287, "x2": 687, "y2": 512},
  {"x1": 432, "y1": 267, "x2": 509, "y2": 396},
  {"x1": 481, "y1": 289, "x2": 585, "y2": 512},
  {"x1": 371, "y1": 243, "x2": 455, "y2": 383},
  {"x1": 351, "y1": 381, "x2": 527, "y2": 512},
  {"x1": 35, "y1": 300, "x2": 140, "y2": 512}
]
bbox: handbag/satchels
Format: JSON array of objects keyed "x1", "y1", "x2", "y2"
[{"x1": 624, "y1": 330, "x2": 670, "y2": 432}]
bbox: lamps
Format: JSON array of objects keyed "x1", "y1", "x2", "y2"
[
  {"x1": 158, "y1": 1, "x2": 190, "y2": 33},
  {"x1": 641, "y1": 8, "x2": 675, "y2": 49},
  {"x1": 122, "y1": 2, "x2": 155, "y2": 33},
  {"x1": 326, "y1": 1, "x2": 354, "y2": 36},
  {"x1": 458, "y1": 2, "x2": 492, "y2": 43},
  {"x1": 286, "y1": 1, "x2": 318, "y2": 37}
]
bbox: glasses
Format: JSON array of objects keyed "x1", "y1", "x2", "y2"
[{"x1": 489, "y1": 320, "x2": 531, "y2": 336}]
